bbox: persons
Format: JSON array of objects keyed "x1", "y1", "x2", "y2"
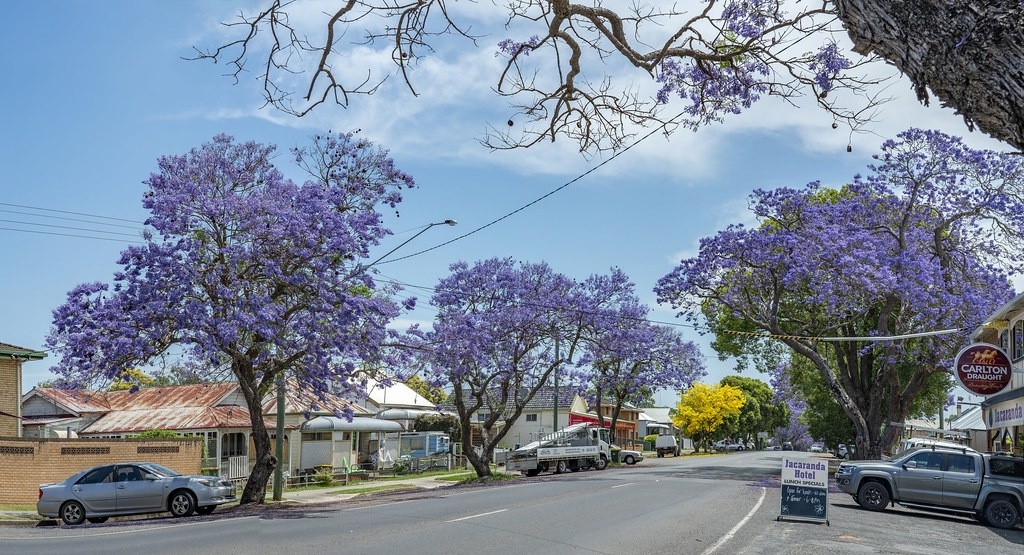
[
  {"x1": 909, "y1": 444, "x2": 937, "y2": 468},
  {"x1": 120, "y1": 472, "x2": 136, "y2": 481},
  {"x1": 724, "y1": 436, "x2": 731, "y2": 454},
  {"x1": 959, "y1": 449, "x2": 968, "y2": 469}
]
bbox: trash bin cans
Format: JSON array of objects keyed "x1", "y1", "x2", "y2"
[{"x1": 611, "y1": 449, "x2": 621, "y2": 465}]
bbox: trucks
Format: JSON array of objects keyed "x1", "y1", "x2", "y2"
[
  {"x1": 656, "y1": 435, "x2": 679, "y2": 458},
  {"x1": 506, "y1": 427, "x2": 610, "y2": 477}
]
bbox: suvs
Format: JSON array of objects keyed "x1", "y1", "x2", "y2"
[{"x1": 833, "y1": 443, "x2": 1024, "y2": 529}]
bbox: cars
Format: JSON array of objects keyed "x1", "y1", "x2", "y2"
[
  {"x1": 782, "y1": 442, "x2": 793, "y2": 451},
  {"x1": 612, "y1": 444, "x2": 643, "y2": 466},
  {"x1": 838, "y1": 445, "x2": 855, "y2": 460},
  {"x1": 746, "y1": 441, "x2": 756, "y2": 451},
  {"x1": 764, "y1": 445, "x2": 781, "y2": 450},
  {"x1": 713, "y1": 438, "x2": 745, "y2": 451},
  {"x1": 810, "y1": 444, "x2": 823, "y2": 452}
]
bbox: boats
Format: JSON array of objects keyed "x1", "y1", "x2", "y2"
[{"x1": 36, "y1": 462, "x2": 236, "y2": 526}]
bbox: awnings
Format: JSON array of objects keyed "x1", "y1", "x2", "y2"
[
  {"x1": 303, "y1": 416, "x2": 405, "y2": 432},
  {"x1": 381, "y1": 408, "x2": 458, "y2": 420}
]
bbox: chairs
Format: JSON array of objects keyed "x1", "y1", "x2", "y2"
[
  {"x1": 939, "y1": 461, "x2": 951, "y2": 471},
  {"x1": 127, "y1": 472, "x2": 135, "y2": 480},
  {"x1": 119, "y1": 473, "x2": 127, "y2": 481}
]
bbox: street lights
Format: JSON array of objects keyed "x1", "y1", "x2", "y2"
[{"x1": 273, "y1": 219, "x2": 459, "y2": 500}]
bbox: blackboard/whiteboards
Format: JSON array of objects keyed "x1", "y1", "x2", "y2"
[{"x1": 780, "y1": 483, "x2": 827, "y2": 521}]
